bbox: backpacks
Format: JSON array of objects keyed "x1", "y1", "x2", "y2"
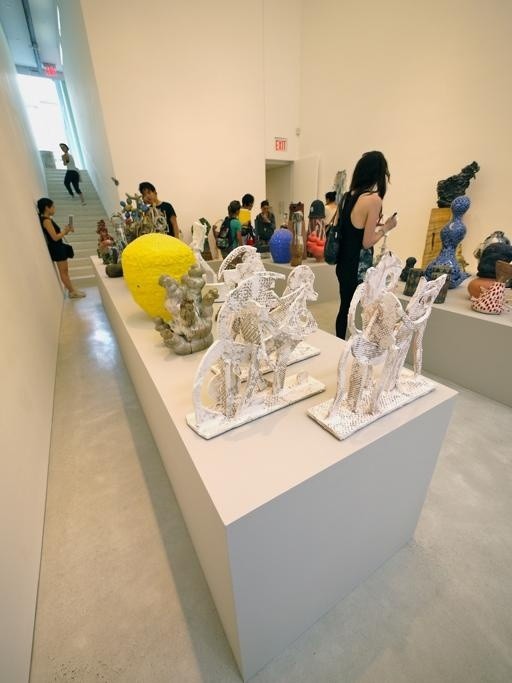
[{"x1": 216, "y1": 216, "x2": 236, "y2": 249}]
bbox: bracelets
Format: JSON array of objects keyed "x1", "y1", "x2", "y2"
[{"x1": 378, "y1": 229, "x2": 386, "y2": 234}]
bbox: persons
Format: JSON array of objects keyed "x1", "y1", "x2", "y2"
[
  {"x1": 59, "y1": 142, "x2": 86, "y2": 205},
  {"x1": 256, "y1": 199, "x2": 276, "y2": 244},
  {"x1": 221, "y1": 200, "x2": 244, "y2": 264},
  {"x1": 237, "y1": 193, "x2": 254, "y2": 244},
  {"x1": 37, "y1": 197, "x2": 86, "y2": 298},
  {"x1": 336, "y1": 150, "x2": 398, "y2": 339},
  {"x1": 324, "y1": 191, "x2": 339, "y2": 228},
  {"x1": 139, "y1": 181, "x2": 178, "y2": 238}
]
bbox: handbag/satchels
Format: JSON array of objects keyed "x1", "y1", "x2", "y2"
[
  {"x1": 324, "y1": 219, "x2": 341, "y2": 265},
  {"x1": 63, "y1": 243, "x2": 74, "y2": 258}
]
